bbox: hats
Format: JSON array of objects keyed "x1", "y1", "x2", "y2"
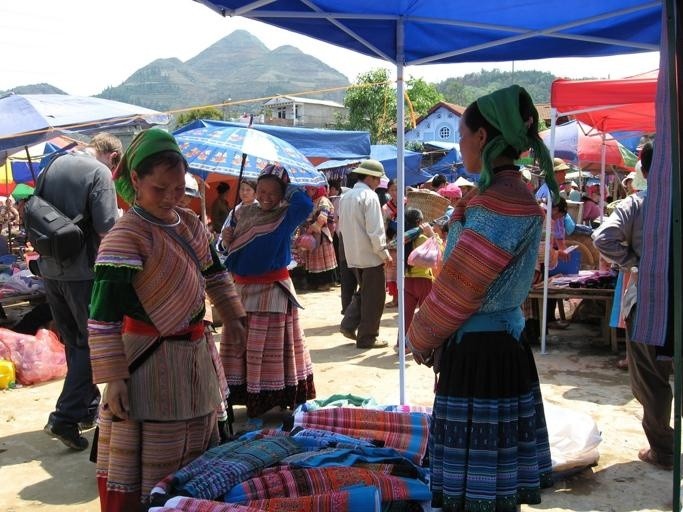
[
  {"x1": 539, "y1": 158, "x2": 570, "y2": 176},
  {"x1": 454, "y1": 177, "x2": 474, "y2": 186},
  {"x1": 622, "y1": 160, "x2": 647, "y2": 190},
  {"x1": 352, "y1": 160, "x2": 384, "y2": 178},
  {"x1": 184, "y1": 173, "x2": 199, "y2": 197}
]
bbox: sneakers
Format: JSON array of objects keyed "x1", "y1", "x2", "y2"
[
  {"x1": 81, "y1": 414, "x2": 97, "y2": 429},
  {"x1": 369, "y1": 339, "x2": 388, "y2": 349},
  {"x1": 545, "y1": 319, "x2": 568, "y2": 346},
  {"x1": 302, "y1": 281, "x2": 341, "y2": 291},
  {"x1": 638, "y1": 448, "x2": 673, "y2": 471},
  {"x1": 617, "y1": 360, "x2": 629, "y2": 371},
  {"x1": 386, "y1": 301, "x2": 398, "y2": 308},
  {"x1": 339, "y1": 325, "x2": 356, "y2": 339},
  {"x1": 44, "y1": 411, "x2": 89, "y2": 451}
]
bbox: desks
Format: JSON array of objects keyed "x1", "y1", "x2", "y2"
[
  {"x1": 0, "y1": 257, "x2": 45, "y2": 303},
  {"x1": 528, "y1": 267, "x2": 619, "y2": 351}
]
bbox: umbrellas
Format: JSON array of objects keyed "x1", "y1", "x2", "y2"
[
  {"x1": 0, "y1": 92, "x2": 172, "y2": 162},
  {"x1": 172, "y1": 123, "x2": 329, "y2": 241},
  {"x1": 540, "y1": 120, "x2": 642, "y2": 195}
]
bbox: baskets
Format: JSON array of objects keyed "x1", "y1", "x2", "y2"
[{"x1": 406, "y1": 190, "x2": 451, "y2": 223}]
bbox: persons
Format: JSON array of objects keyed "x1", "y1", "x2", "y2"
[
  {"x1": 86, "y1": 125, "x2": 245, "y2": 511},
  {"x1": 32, "y1": 130, "x2": 127, "y2": 450},
  {"x1": 1, "y1": 135, "x2": 673, "y2": 370},
  {"x1": 406, "y1": 85, "x2": 556, "y2": 510},
  {"x1": 591, "y1": 139, "x2": 676, "y2": 473},
  {"x1": 217, "y1": 163, "x2": 314, "y2": 425}
]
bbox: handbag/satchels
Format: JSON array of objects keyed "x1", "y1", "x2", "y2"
[{"x1": 23, "y1": 195, "x2": 86, "y2": 264}]
